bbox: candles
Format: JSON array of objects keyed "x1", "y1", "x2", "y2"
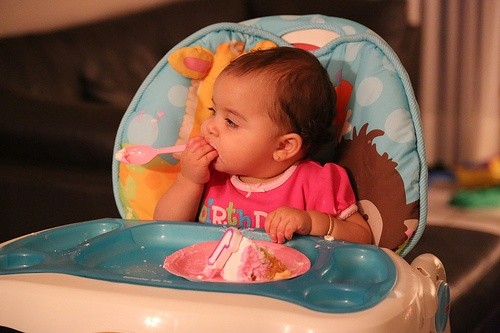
[{"x1": 202, "y1": 226, "x2": 243, "y2": 279}]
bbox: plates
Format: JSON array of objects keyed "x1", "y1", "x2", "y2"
[{"x1": 164, "y1": 240, "x2": 312, "y2": 284}]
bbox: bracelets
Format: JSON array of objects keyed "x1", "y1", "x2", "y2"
[{"x1": 325, "y1": 212, "x2": 334, "y2": 236}]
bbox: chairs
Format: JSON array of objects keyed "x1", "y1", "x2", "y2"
[{"x1": 0, "y1": 14, "x2": 451, "y2": 333}]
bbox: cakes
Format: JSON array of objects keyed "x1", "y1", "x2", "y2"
[{"x1": 204, "y1": 227, "x2": 291, "y2": 282}]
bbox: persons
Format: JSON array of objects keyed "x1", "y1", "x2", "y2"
[{"x1": 154, "y1": 46, "x2": 372, "y2": 246}]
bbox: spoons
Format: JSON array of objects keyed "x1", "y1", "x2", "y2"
[{"x1": 116, "y1": 144, "x2": 187, "y2": 165}]
